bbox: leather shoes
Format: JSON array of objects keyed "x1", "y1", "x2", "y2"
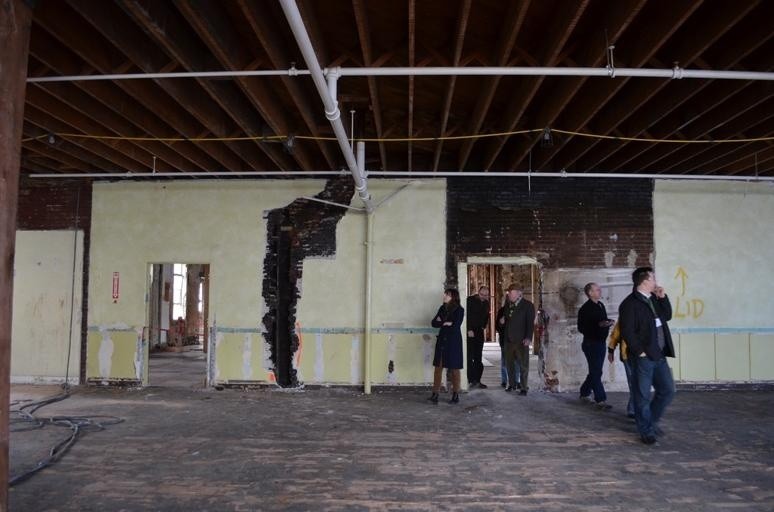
[
  {"x1": 469, "y1": 382, "x2": 486, "y2": 389},
  {"x1": 448, "y1": 392, "x2": 459, "y2": 404},
  {"x1": 427, "y1": 392, "x2": 439, "y2": 403},
  {"x1": 501, "y1": 383, "x2": 528, "y2": 396}
]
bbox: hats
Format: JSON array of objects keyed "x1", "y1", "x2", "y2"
[{"x1": 504, "y1": 284, "x2": 521, "y2": 291}]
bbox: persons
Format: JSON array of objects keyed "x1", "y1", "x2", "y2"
[
  {"x1": 467, "y1": 286, "x2": 490, "y2": 389},
  {"x1": 504, "y1": 284, "x2": 535, "y2": 397},
  {"x1": 577, "y1": 283, "x2": 615, "y2": 410},
  {"x1": 495, "y1": 294, "x2": 520, "y2": 388},
  {"x1": 607, "y1": 320, "x2": 635, "y2": 417},
  {"x1": 426, "y1": 289, "x2": 463, "y2": 403},
  {"x1": 618, "y1": 268, "x2": 676, "y2": 444}
]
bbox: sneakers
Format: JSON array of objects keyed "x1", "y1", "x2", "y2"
[
  {"x1": 628, "y1": 413, "x2": 664, "y2": 444},
  {"x1": 596, "y1": 401, "x2": 612, "y2": 409},
  {"x1": 579, "y1": 395, "x2": 595, "y2": 405}
]
